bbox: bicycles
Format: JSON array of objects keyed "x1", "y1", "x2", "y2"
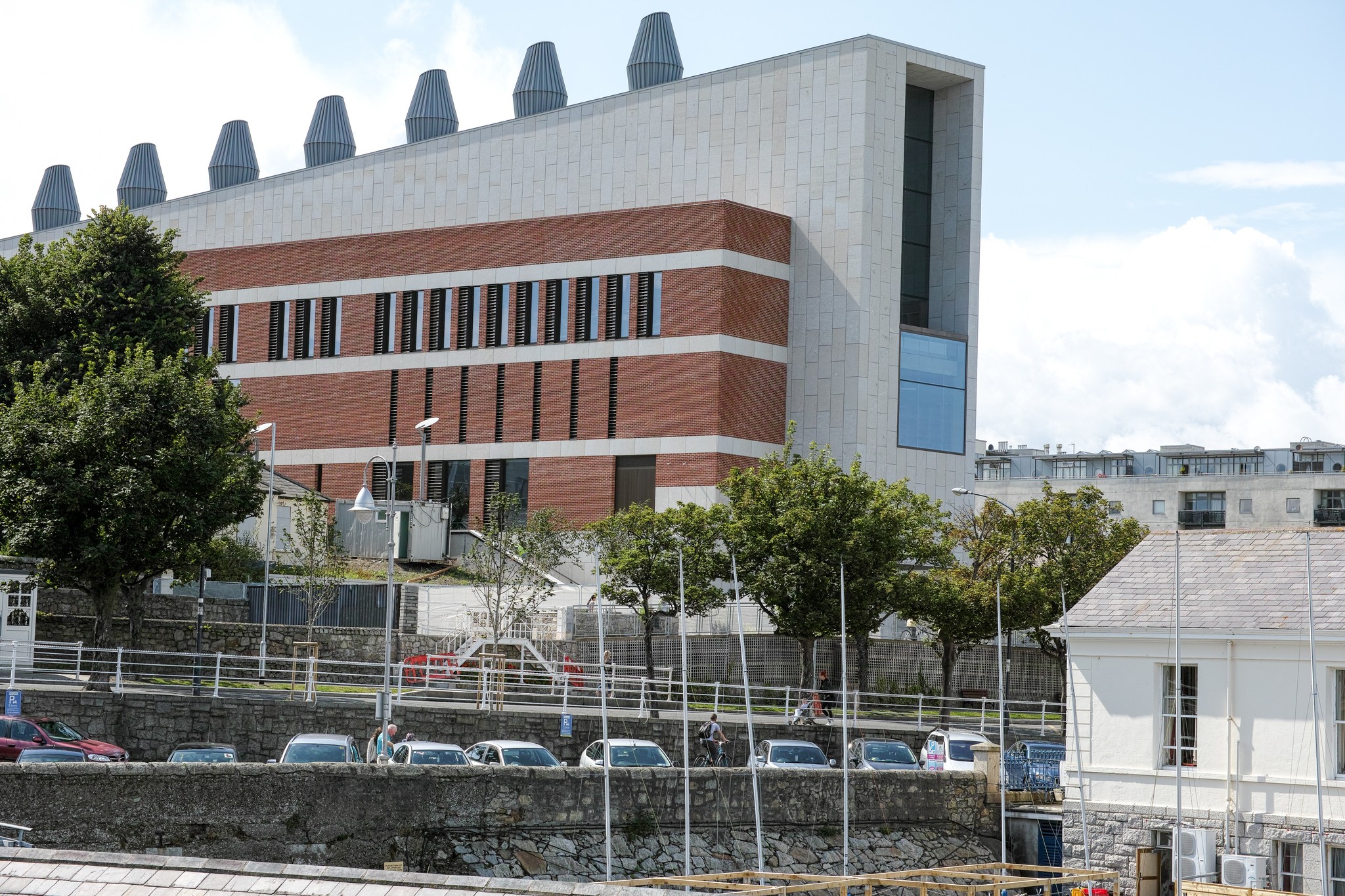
[{"x1": 692, "y1": 741, "x2": 733, "y2": 768}]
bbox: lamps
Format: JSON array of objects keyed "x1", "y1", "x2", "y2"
[{"x1": 1167, "y1": 833, "x2": 1173, "y2": 841}]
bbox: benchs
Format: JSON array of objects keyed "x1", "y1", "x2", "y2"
[{"x1": 962, "y1": 689, "x2": 995, "y2": 710}]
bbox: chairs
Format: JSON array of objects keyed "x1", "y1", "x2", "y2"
[
  {"x1": 23, "y1": 724, "x2": 35, "y2": 741},
  {"x1": 778, "y1": 750, "x2": 789, "y2": 757},
  {"x1": 443, "y1": 754, "x2": 456, "y2": 765},
  {"x1": 12, "y1": 723, "x2": 22, "y2": 740},
  {"x1": 636, "y1": 749, "x2": 652, "y2": 763},
  {"x1": 487, "y1": 751, "x2": 509, "y2": 765},
  {"x1": 612, "y1": 749, "x2": 618, "y2": 762},
  {"x1": 964, "y1": 744, "x2": 974, "y2": 759},
  {"x1": 864, "y1": 746, "x2": 873, "y2": 758},
  {"x1": 216, "y1": 756, "x2": 230, "y2": 763},
  {"x1": 518, "y1": 751, "x2": 536, "y2": 766},
  {"x1": 886, "y1": 749, "x2": 901, "y2": 761}
]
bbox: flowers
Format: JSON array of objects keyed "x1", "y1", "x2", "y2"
[{"x1": 1316, "y1": 504, "x2": 1327, "y2": 509}]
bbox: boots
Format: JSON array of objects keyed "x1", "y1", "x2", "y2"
[
  {"x1": 826, "y1": 720, "x2": 833, "y2": 726},
  {"x1": 826, "y1": 717, "x2": 830, "y2": 724}
]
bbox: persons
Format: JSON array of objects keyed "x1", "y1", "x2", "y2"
[
  {"x1": 587, "y1": 595, "x2": 596, "y2": 613},
  {"x1": 700, "y1": 714, "x2": 729, "y2": 767},
  {"x1": 402, "y1": 733, "x2": 419, "y2": 742},
  {"x1": 800, "y1": 698, "x2": 809, "y2": 710},
  {"x1": 367, "y1": 727, "x2": 383, "y2": 764},
  {"x1": 594, "y1": 651, "x2": 612, "y2": 698},
  {"x1": 377, "y1": 724, "x2": 397, "y2": 764},
  {"x1": 810, "y1": 670, "x2": 833, "y2": 726}
]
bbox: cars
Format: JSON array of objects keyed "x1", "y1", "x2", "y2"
[
  {"x1": 166, "y1": 741, "x2": 241, "y2": 763},
  {"x1": 388, "y1": 741, "x2": 473, "y2": 766},
  {"x1": 16, "y1": 745, "x2": 90, "y2": 762},
  {"x1": 747, "y1": 739, "x2": 836, "y2": 769},
  {"x1": 267, "y1": 733, "x2": 363, "y2": 763},
  {"x1": 463, "y1": 740, "x2": 569, "y2": 766},
  {"x1": 580, "y1": 739, "x2": 674, "y2": 769},
  {"x1": 840, "y1": 737, "x2": 926, "y2": 770},
  {"x1": 1003, "y1": 740, "x2": 1066, "y2": 788},
  {"x1": 0, "y1": 715, "x2": 130, "y2": 765}
]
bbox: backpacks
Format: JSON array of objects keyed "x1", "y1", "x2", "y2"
[{"x1": 698, "y1": 721, "x2": 715, "y2": 739}]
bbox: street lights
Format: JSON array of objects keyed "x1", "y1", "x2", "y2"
[
  {"x1": 950, "y1": 486, "x2": 1016, "y2": 737},
  {"x1": 345, "y1": 439, "x2": 400, "y2": 766},
  {"x1": 414, "y1": 418, "x2": 441, "y2": 500},
  {"x1": 252, "y1": 422, "x2": 277, "y2": 688}
]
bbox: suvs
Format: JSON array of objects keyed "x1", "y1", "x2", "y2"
[{"x1": 920, "y1": 728, "x2": 1010, "y2": 785}]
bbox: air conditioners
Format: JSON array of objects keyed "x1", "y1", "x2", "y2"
[
  {"x1": 1172, "y1": 827, "x2": 1216, "y2": 883},
  {"x1": 1221, "y1": 854, "x2": 1267, "y2": 890}
]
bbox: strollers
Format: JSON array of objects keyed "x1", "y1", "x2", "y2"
[{"x1": 788, "y1": 693, "x2": 817, "y2": 725}]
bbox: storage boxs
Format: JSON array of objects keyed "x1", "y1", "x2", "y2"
[{"x1": 1072, "y1": 888, "x2": 1125, "y2": 896}]
